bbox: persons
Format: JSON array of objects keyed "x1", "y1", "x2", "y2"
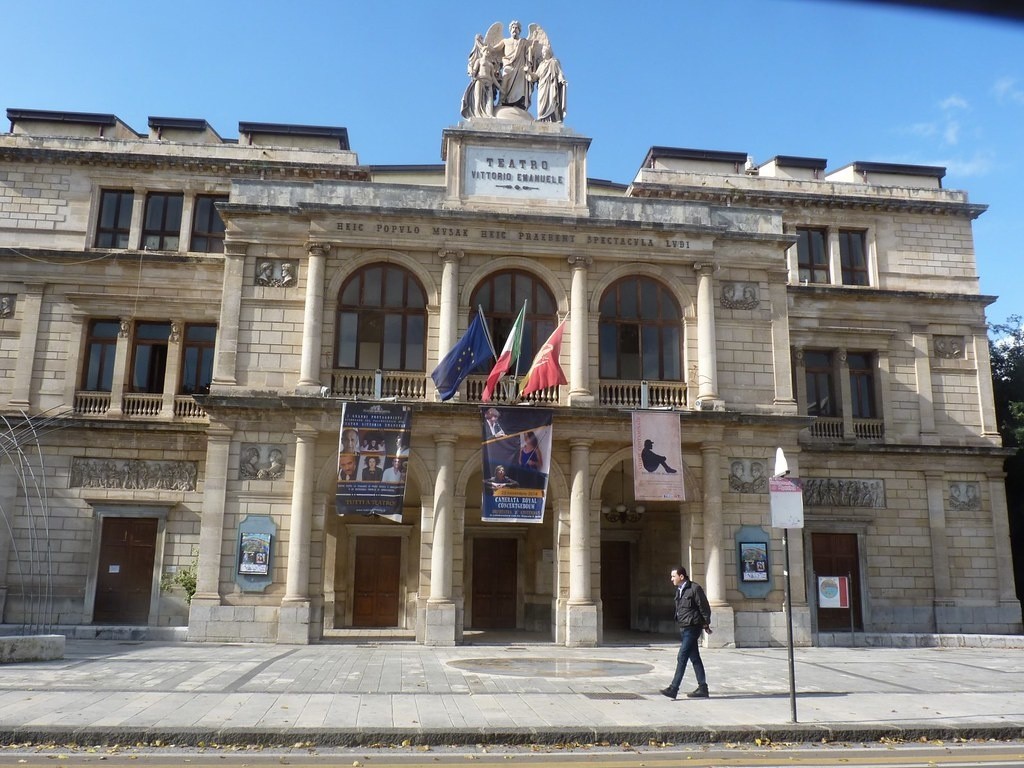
[
  {"x1": 483, "y1": 408, "x2": 508, "y2": 444},
  {"x1": 523, "y1": 46, "x2": 568, "y2": 123},
  {"x1": 339, "y1": 456, "x2": 357, "y2": 480},
  {"x1": 341, "y1": 429, "x2": 358, "y2": 454},
  {"x1": 461, "y1": 46, "x2": 505, "y2": 118},
  {"x1": 659, "y1": 566, "x2": 712, "y2": 699},
  {"x1": 474, "y1": 20, "x2": 539, "y2": 111},
  {"x1": 360, "y1": 433, "x2": 409, "y2": 482},
  {"x1": 484, "y1": 465, "x2": 519, "y2": 489},
  {"x1": 519, "y1": 432, "x2": 542, "y2": 470}
]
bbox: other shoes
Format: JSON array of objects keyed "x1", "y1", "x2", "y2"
[{"x1": 666, "y1": 469, "x2": 677, "y2": 473}]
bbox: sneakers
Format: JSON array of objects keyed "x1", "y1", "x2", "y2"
[
  {"x1": 660, "y1": 685, "x2": 679, "y2": 700},
  {"x1": 687, "y1": 683, "x2": 709, "y2": 699}
]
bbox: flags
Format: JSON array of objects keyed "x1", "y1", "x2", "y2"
[
  {"x1": 431, "y1": 313, "x2": 492, "y2": 401},
  {"x1": 519, "y1": 321, "x2": 568, "y2": 396},
  {"x1": 481, "y1": 308, "x2": 524, "y2": 403}
]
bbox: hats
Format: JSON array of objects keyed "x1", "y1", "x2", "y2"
[{"x1": 644, "y1": 439, "x2": 653, "y2": 447}]
